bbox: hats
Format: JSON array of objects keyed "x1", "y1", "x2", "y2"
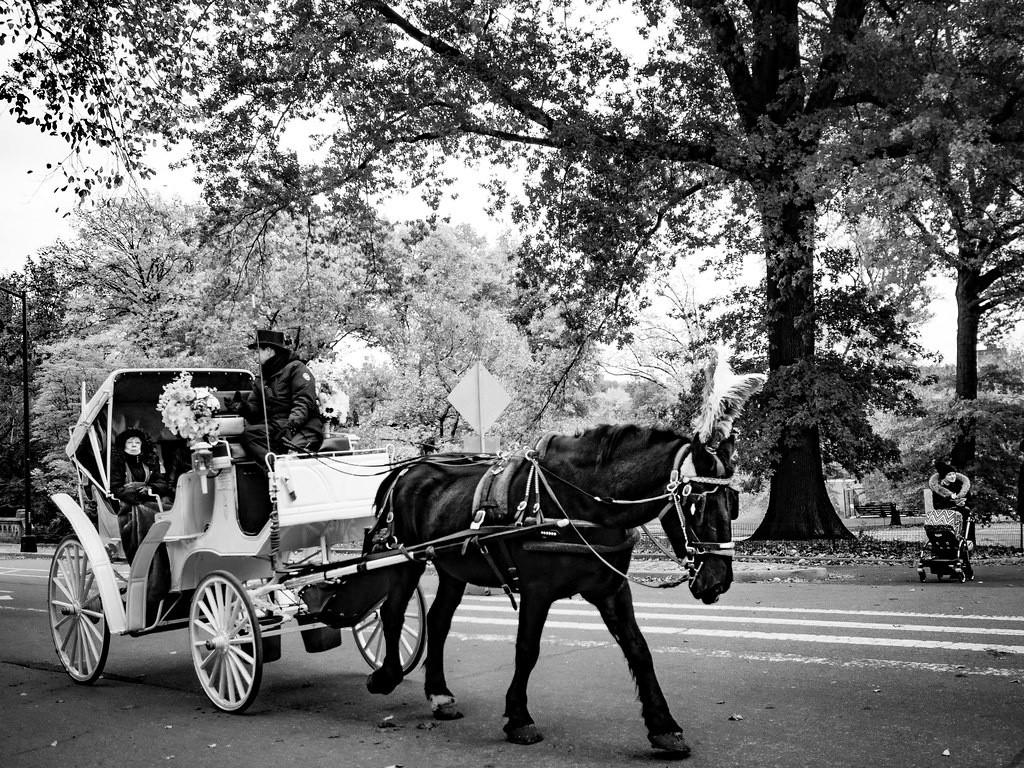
[
  {"x1": 247, "y1": 330, "x2": 291, "y2": 353},
  {"x1": 114, "y1": 428, "x2": 148, "y2": 451}
]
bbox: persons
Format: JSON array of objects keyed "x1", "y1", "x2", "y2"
[
  {"x1": 928, "y1": 462, "x2": 976, "y2": 579},
  {"x1": 222, "y1": 327, "x2": 325, "y2": 476},
  {"x1": 109, "y1": 424, "x2": 173, "y2": 568}
]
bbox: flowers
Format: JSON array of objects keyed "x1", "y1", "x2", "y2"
[
  {"x1": 156, "y1": 371, "x2": 220, "y2": 448},
  {"x1": 315, "y1": 377, "x2": 352, "y2": 439}
]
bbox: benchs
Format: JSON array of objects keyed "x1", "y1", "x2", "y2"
[
  {"x1": 855, "y1": 503, "x2": 902, "y2": 518},
  {"x1": 214, "y1": 414, "x2": 351, "y2": 517}
]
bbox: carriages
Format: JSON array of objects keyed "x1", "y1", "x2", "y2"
[{"x1": 45, "y1": 365, "x2": 741, "y2": 760}]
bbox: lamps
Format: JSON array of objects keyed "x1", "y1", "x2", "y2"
[{"x1": 192, "y1": 442, "x2": 213, "y2": 495}]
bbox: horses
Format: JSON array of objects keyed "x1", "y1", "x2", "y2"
[{"x1": 343, "y1": 423, "x2": 741, "y2": 759}]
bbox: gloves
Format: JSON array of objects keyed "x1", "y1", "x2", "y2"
[
  {"x1": 223, "y1": 391, "x2": 248, "y2": 420},
  {"x1": 275, "y1": 428, "x2": 292, "y2": 441},
  {"x1": 136, "y1": 486, "x2": 152, "y2": 494}
]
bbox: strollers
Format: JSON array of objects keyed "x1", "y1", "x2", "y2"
[{"x1": 917, "y1": 505, "x2": 975, "y2": 582}]
